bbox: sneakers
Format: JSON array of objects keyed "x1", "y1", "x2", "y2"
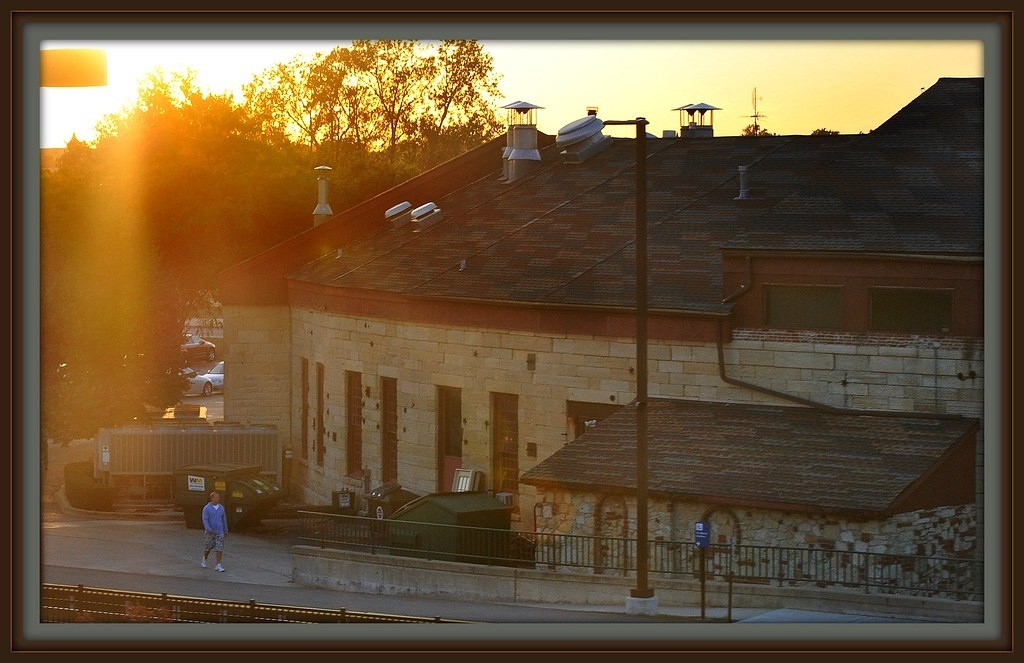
[
  {"x1": 201, "y1": 559, "x2": 207, "y2": 567},
  {"x1": 214, "y1": 564, "x2": 225, "y2": 572}
]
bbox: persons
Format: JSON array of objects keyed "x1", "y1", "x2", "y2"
[{"x1": 202, "y1": 491, "x2": 228, "y2": 572}]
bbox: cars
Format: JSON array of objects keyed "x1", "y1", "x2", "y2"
[
  {"x1": 181, "y1": 335, "x2": 216, "y2": 367},
  {"x1": 201, "y1": 360, "x2": 224, "y2": 392},
  {"x1": 177, "y1": 366, "x2": 213, "y2": 396}
]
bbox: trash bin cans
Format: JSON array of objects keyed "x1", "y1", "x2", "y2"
[
  {"x1": 175, "y1": 463, "x2": 283, "y2": 532},
  {"x1": 367, "y1": 483, "x2": 515, "y2": 564}
]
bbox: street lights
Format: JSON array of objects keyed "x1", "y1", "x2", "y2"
[{"x1": 556, "y1": 115, "x2": 661, "y2": 616}]
